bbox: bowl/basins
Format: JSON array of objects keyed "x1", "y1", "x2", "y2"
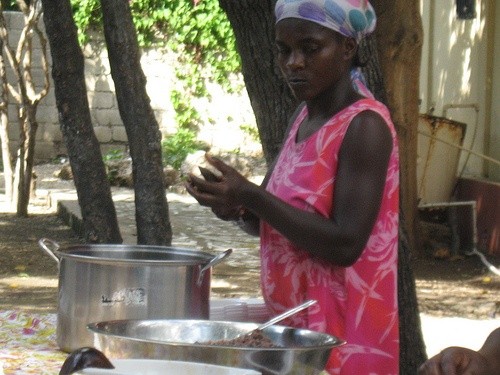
[
  {"x1": 79, "y1": 359, "x2": 262, "y2": 375},
  {"x1": 86, "y1": 316, "x2": 346, "y2": 375}
]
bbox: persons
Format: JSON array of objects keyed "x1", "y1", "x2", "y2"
[
  {"x1": 182, "y1": 0, "x2": 400, "y2": 375},
  {"x1": 415, "y1": 325, "x2": 500, "y2": 375}
]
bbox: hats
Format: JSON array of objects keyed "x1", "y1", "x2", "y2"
[{"x1": 274, "y1": 0, "x2": 376, "y2": 42}]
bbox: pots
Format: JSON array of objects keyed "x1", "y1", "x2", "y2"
[{"x1": 38, "y1": 237, "x2": 231, "y2": 355}]
